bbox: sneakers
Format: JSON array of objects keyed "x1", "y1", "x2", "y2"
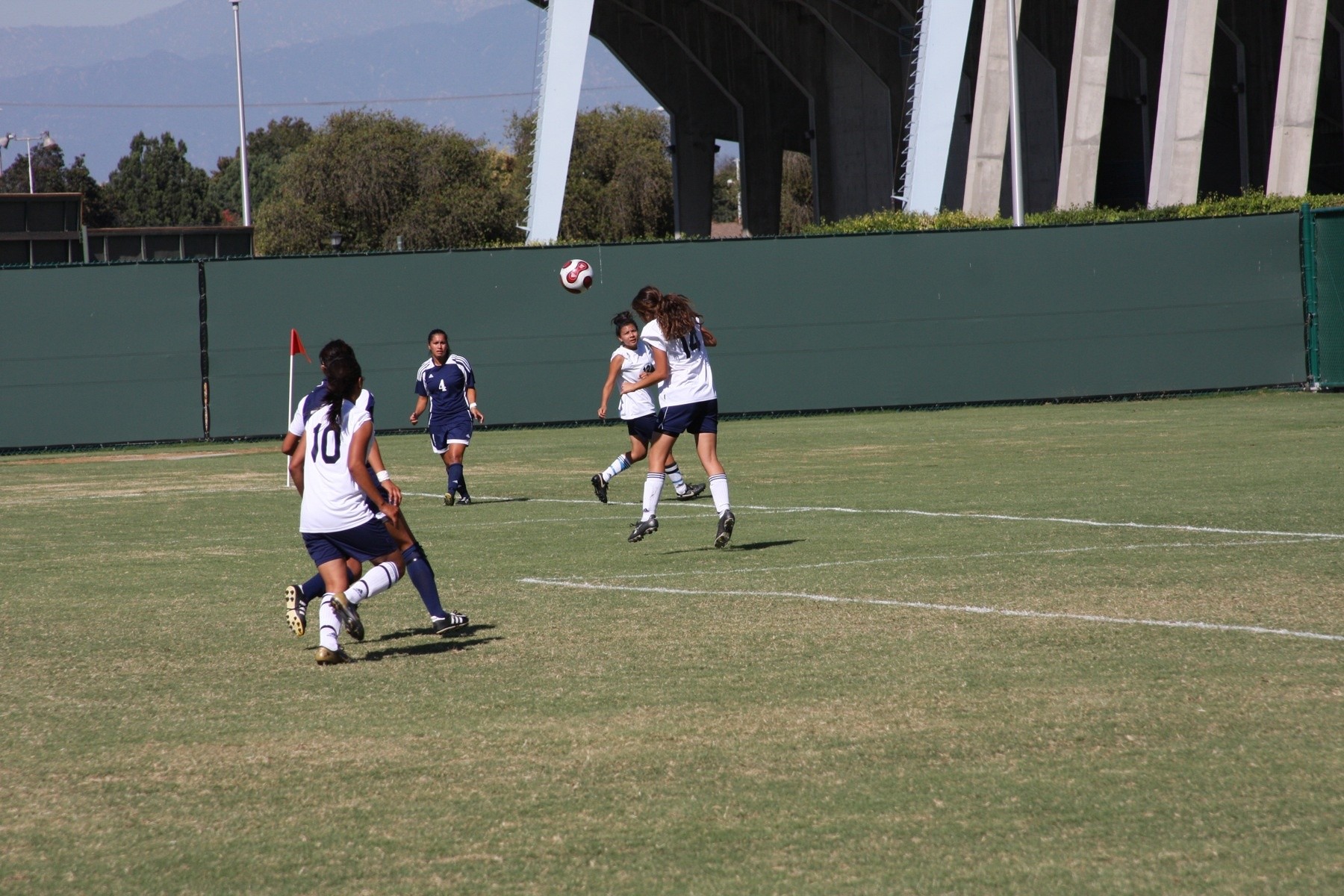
[
  {"x1": 316, "y1": 644, "x2": 358, "y2": 665},
  {"x1": 676, "y1": 483, "x2": 706, "y2": 500},
  {"x1": 628, "y1": 515, "x2": 658, "y2": 543},
  {"x1": 330, "y1": 592, "x2": 364, "y2": 640},
  {"x1": 591, "y1": 473, "x2": 609, "y2": 504},
  {"x1": 714, "y1": 509, "x2": 734, "y2": 549},
  {"x1": 284, "y1": 585, "x2": 308, "y2": 636},
  {"x1": 444, "y1": 493, "x2": 455, "y2": 506},
  {"x1": 456, "y1": 497, "x2": 471, "y2": 505},
  {"x1": 430, "y1": 610, "x2": 469, "y2": 634}
]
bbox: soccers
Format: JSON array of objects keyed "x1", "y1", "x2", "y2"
[{"x1": 560, "y1": 259, "x2": 593, "y2": 294}]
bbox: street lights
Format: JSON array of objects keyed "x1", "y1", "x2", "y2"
[{"x1": 0, "y1": 132, "x2": 58, "y2": 193}]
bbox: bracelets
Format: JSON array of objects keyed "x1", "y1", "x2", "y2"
[
  {"x1": 376, "y1": 469, "x2": 391, "y2": 482},
  {"x1": 469, "y1": 402, "x2": 477, "y2": 409}
]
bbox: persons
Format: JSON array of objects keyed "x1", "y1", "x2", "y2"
[
  {"x1": 591, "y1": 310, "x2": 706, "y2": 504},
  {"x1": 626, "y1": 286, "x2": 735, "y2": 549},
  {"x1": 284, "y1": 339, "x2": 469, "y2": 635},
  {"x1": 289, "y1": 355, "x2": 406, "y2": 666},
  {"x1": 410, "y1": 328, "x2": 485, "y2": 506}
]
